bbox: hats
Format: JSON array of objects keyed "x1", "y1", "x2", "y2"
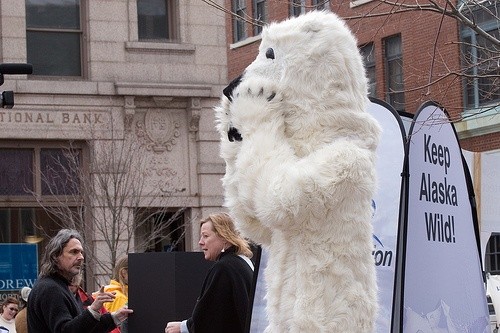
[{"x1": 21, "y1": 287, "x2": 32, "y2": 303}]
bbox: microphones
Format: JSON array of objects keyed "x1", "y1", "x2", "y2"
[{"x1": 0, "y1": 63, "x2": 32, "y2": 75}]
